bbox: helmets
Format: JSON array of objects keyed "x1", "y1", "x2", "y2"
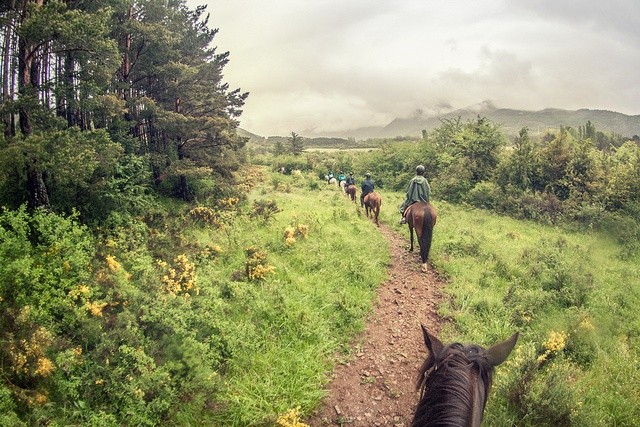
[
  {"x1": 415, "y1": 165, "x2": 425, "y2": 173},
  {"x1": 365, "y1": 173, "x2": 371, "y2": 177}
]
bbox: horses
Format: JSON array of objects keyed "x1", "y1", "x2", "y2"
[
  {"x1": 346, "y1": 185, "x2": 356, "y2": 203},
  {"x1": 324, "y1": 174, "x2": 336, "y2": 185},
  {"x1": 410, "y1": 324, "x2": 519, "y2": 427},
  {"x1": 364, "y1": 192, "x2": 381, "y2": 226},
  {"x1": 340, "y1": 181, "x2": 346, "y2": 195},
  {"x1": 405, "y1": 201, "x2": 437, "y2": 273}
]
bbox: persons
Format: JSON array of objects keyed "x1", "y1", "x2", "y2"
[
  {"x1": 327, "y1": 171, "x2": 333, "y2": 180},
  {"x1": 338, "y1": 170, "x2": 346, "y2": 181},
  {"x1": 398, "y1": 164, "x2": 431, "y2": 224},
  {"x1": 345, "y1": 170, "x2": 355, "y2": 189},
  {"x1": 359, "y1": 172, "x2": 374, "y2": 208}
]
